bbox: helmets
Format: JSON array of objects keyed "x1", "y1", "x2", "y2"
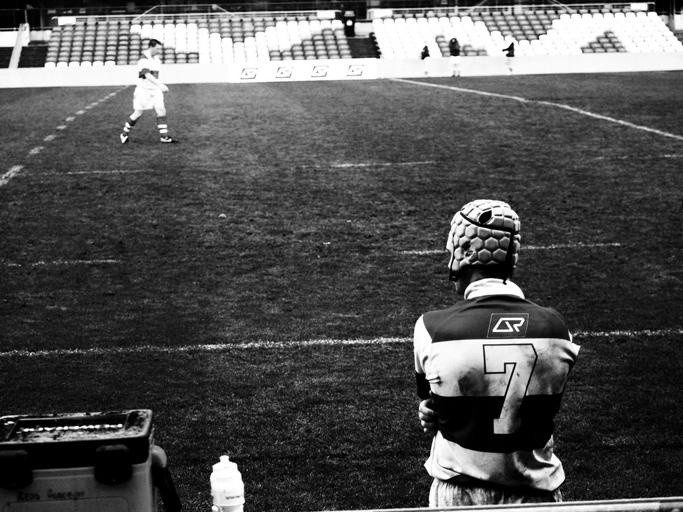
[{"x1": 444, "y1": 197, "x2": 525, "y2": 274}]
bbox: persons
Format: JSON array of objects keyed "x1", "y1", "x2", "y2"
[
  {"x1": 408, "y1": 196, "x2": 584, "y2": 506},
  {"x1": 445, "y1": 37, "x2": 464, "y2": 77},
  {"x1": 415, "y1": 44, "x2": 434, "y2": 75},
  {"x1": 500, "y1": 40, "x2": 515, "y2": 75},
  {"x1": 117, "y1": 36, "x2": 175, "y2": 144}
]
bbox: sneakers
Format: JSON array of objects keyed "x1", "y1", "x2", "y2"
[
  {"x1": 160, "y1": 135, "x2": 180, "y2": 144},
  {"x1": 119, "y1": 132, "x2": 130, "y2": 144}
]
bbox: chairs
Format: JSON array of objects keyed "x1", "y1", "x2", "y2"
[
  {"x1": 44, "y1": 8, "x2": 354, "y2": 71},
  {"x1": 365, "y1": 3, "x2": 683, "y2": 60}
]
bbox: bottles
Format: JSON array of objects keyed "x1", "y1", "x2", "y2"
[{"x1": 209, "y1": 455, "x2": 245, "y2": 512}]
